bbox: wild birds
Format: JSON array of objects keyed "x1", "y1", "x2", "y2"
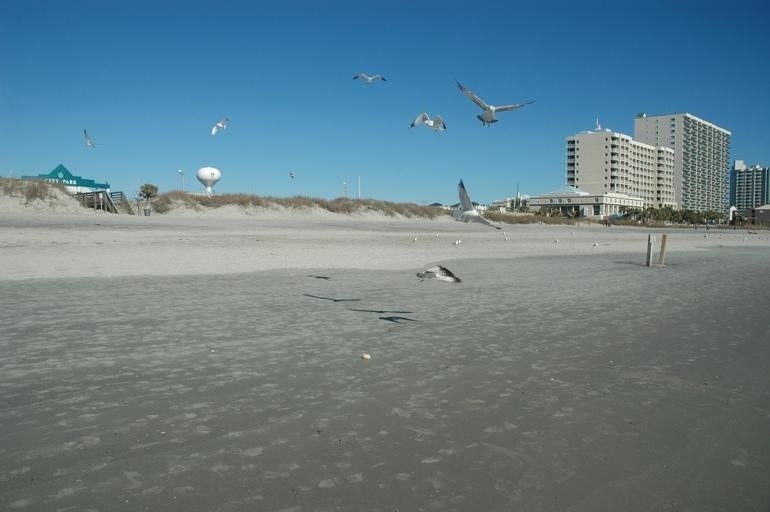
[
  {"x1": 81, "y1": 128, "x2": 100, "y2": 148},
  {"x1": 353, "y1": 72, "x2": 386, "y2": 85},
  {"x1": 209, "y1": 117, "x2": 231, "y2": 136},
  {"x1": 289, "y1": 172, "x2": 295, "y2": 179},
  {"x1": 456, "y1": 81, "x2": 536, "y2": 128},
  {"x1": 416, "y1": 264, "x2": 461, "y2": 285},
  {"x1": 408, "y1": 112, "x2": 447, "y2": 134},
  {"x1": 453, "y1": 176, "x2": 500, "y2": 232}
]
